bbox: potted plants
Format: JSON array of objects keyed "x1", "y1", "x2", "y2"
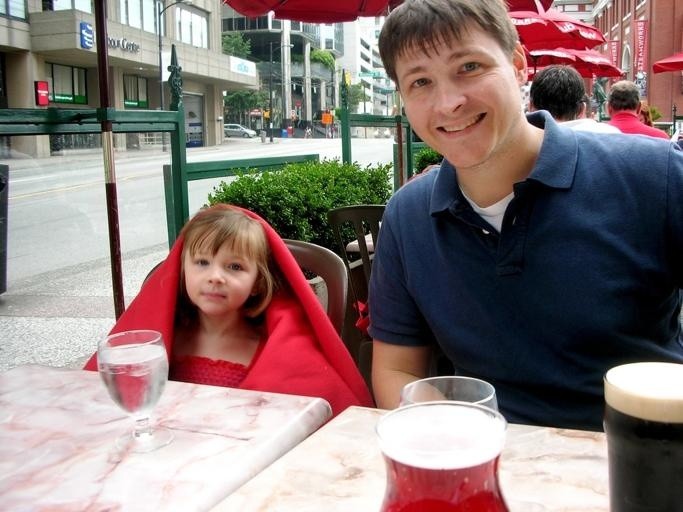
[{"x1": 200, "y1": 156, "x2": 394, "y2": 365}]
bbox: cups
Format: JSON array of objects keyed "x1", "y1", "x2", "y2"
[
  {"x1": 601, "y1": 360, "x2": 682, "y2": 512},
  {"x1": 371, "y1": 401, "x2": 513, "y2": 512},
  {"x1": 396, "y1": 376, "x2": 498, "y2": 414}
]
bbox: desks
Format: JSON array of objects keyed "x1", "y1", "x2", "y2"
[
  {"x1": 211, "y1": 405, "x2": 610, "y2": 512},
  {"x1": 0, "y1": 362, "x2": 333, "y2": 512}
]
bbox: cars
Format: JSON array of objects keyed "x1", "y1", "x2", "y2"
[{"x1": 373, "y1": 127, "x2": 390, "y2": 138}]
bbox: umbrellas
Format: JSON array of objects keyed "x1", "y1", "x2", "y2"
[
  {"x1": 645, "y1": 52, "x2": 683, "y2": 75},
  {"x1": 507, "y1": 7, "x2": 628, "y2": 79},
  {"x1": 222, "y1": 1, "x2": 551, "y2": 28}
]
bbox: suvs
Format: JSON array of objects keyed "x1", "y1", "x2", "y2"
[{"x1": 224, "y1": 124, "x2": 256, "y2": 138}]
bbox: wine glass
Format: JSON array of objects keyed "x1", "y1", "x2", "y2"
[{"x1": 95, "y1": 328, "x2": 176, "y2": 456}]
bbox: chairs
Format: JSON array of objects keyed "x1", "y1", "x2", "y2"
[
  {"x1": 142, "y1": 239, "x2": 349, "y2": 339},
  {"x1": 326, "y1": 205, "x2": 388, "y2": 341}
]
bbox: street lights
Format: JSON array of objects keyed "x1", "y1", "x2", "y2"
[{"x1": 269, "y1": 43, "x2": 295, "y2": 143}]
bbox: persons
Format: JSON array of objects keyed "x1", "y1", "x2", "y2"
[
  {"x1": 528, "y1": 64, "x2": 587, "y2": 127},
  {"x1": 603, "y1": 77, "x2": 670, "y2": 140},
  {"x1": 369, "y1": 1, "x2": 682, "y2": 439},
  {"x1": 82, "y1": 202, "x2": 376, "y2": 428},
  {"x1": 637, "y1": 100, "x2": 652, "y2": 125}
]
